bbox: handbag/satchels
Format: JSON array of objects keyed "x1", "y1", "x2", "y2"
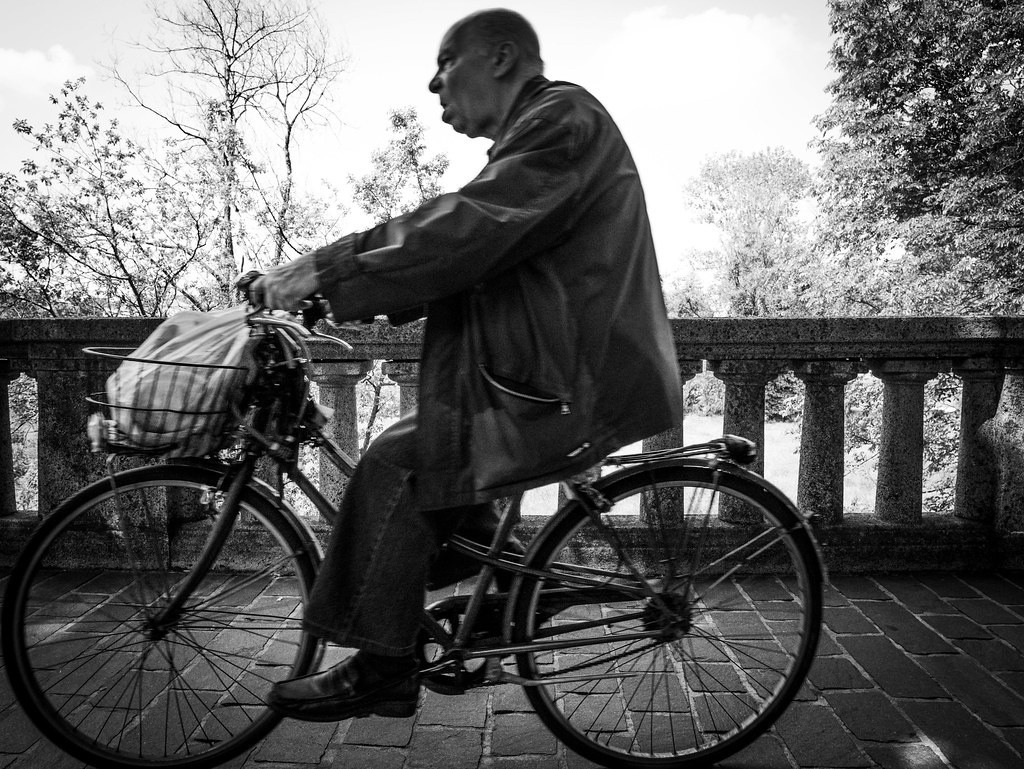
[{"x1": 104, "y1": 300, "x2": 259, "y2": 446}]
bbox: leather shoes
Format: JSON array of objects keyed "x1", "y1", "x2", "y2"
[
  {"x1": 268, "y1": 657, "x2": 420, "y2": 722},
  {"x1": 470, "y1": 577, "x2": 562, "y2": 631}
]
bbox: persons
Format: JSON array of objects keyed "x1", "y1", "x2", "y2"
[{"x1": 250, "y1": 8, "x2": 683, "y2": 724}]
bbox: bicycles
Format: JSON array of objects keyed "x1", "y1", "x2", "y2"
[{"x1": 0, "y1": 268, "x2": 825, "y2": 769}]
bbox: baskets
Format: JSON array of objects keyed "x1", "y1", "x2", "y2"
[{"x1": 82, "y1": 347, "x2": 249, "y2": 458}]
